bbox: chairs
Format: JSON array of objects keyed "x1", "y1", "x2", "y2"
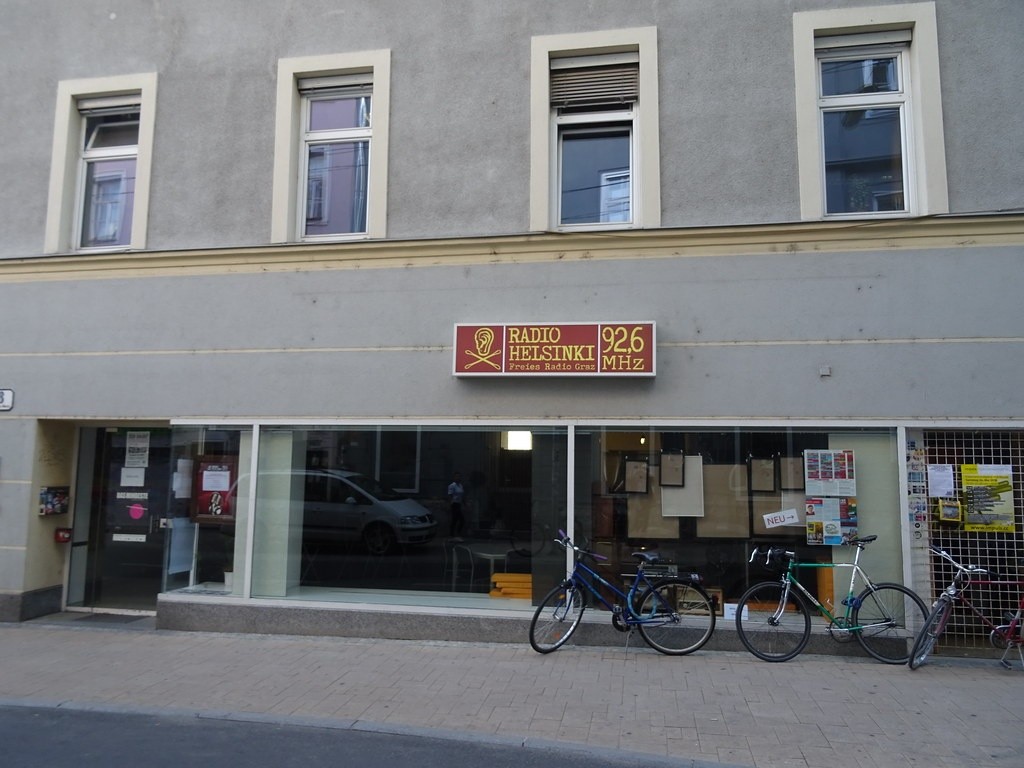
[{"x1": 442, "y1": 535, "x2": 532, "y2": 593}]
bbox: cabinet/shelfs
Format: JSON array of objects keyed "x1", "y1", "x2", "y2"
[{"x1": 593, "y1": 492, "x2": 725, "y2": 618}]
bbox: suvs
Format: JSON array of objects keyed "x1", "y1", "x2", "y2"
[{"x1": 219, "y1": 470, "x2": 439, "y2": 555}]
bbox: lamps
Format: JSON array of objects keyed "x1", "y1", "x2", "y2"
[
  {"x1": 640, "y1": 435, "x2": 646, "y2": 445},
  {"x1": 500, "y1": 431, "x2": 533, "y2": 451}
]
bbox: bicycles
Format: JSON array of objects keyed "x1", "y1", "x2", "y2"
[
  {"x1": 736, "y1": 535, "x2": 930, "y2": 666},
  {"x1": 529, "y1": 529, "x2": 716, "y2": 661},
  {"x1": 910, "y1": 544, "x2": 1024, "y2": 671}
]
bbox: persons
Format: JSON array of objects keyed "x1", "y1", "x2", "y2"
[
  {"x1": 806, "y1": 505, "x2": 815, "y2": 516},
  {"x1": 448, "y1": 473, "x2": 465, "y2": 538}
]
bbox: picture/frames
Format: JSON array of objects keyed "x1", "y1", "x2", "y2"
[
  {"x1": 749, "y1": 456, "x2": 776, "y2": 494},
  {"x1": 624, "y1": 458, "x2": 650, "y2": 495},
  {"x1": 779, "y1": 455, "x2": 806, "y2": 492},
  {"x1": 659, "y1": 451, "x2": 685, "y2": 488}
]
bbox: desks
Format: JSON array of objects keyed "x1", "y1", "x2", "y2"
[{"x1": 470, "y1": 542, "x2": 557, "y2": 594}]
